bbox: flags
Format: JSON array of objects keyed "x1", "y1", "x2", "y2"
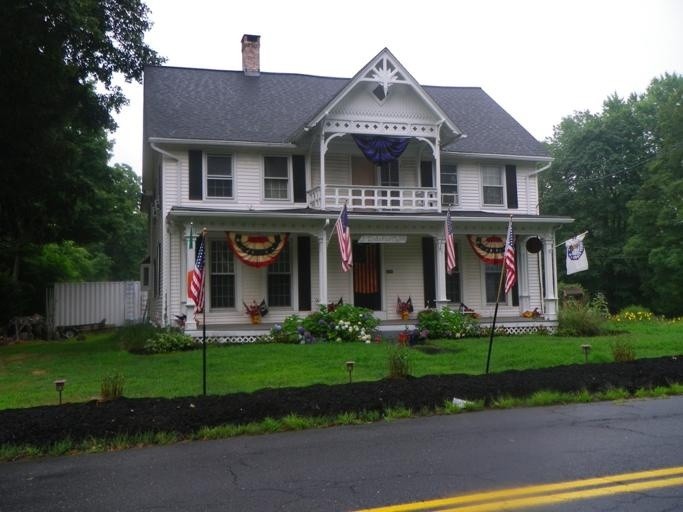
[
  {"x1": 188, "y1": 229, "x2": 207, "y2": 322},
  {"x1": 395, "y1": 294, "x2": 413, "y2": 314},
  {"x1": 334, "y1": 202, "x2": 354, "y2": 273},
  {"x1": 240, "y1": 297, "x2": 269, "y2": 318},
  {"x1": 502, "y1": 217, "x2": 517, "y2": 296},
  {"x1": 459, "y1": 302, "x2": 468, "y2": 311},
  {"x1": 444, "y1": 207, "x2": 456, "y2": 277},
  {"x1": 564, "y1": 232, "x2": 589, "y2": 276}
]
